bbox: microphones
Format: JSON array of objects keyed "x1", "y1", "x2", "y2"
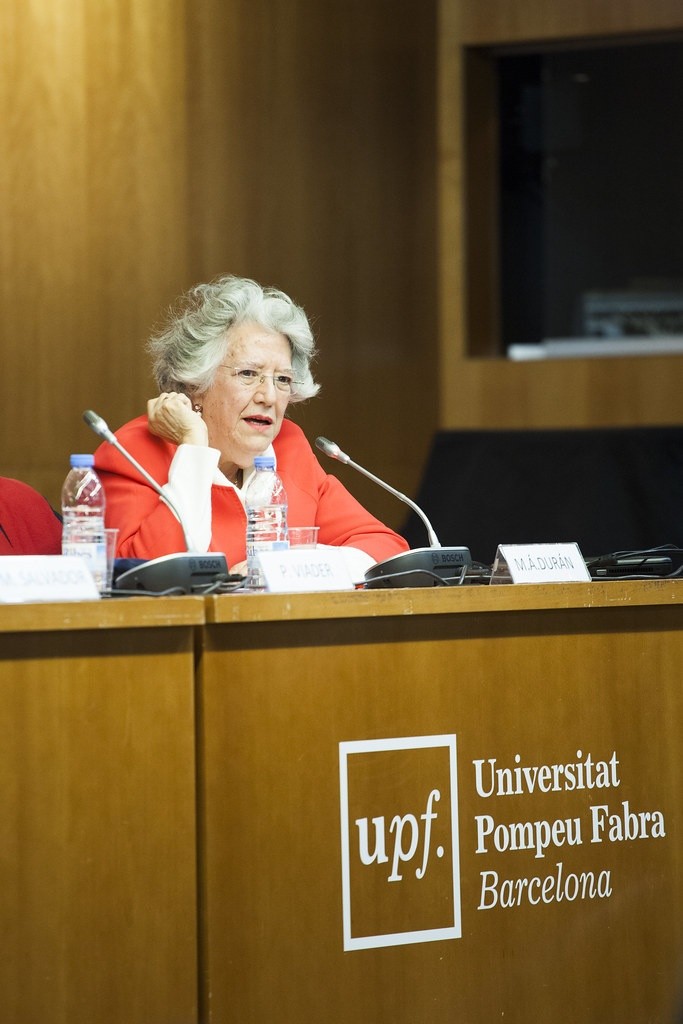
[
  {"x1": 82, "y1": 409, "x2": 230, "y2": 594},
  {"x1": 314, "y1": 434, "x2": 473, "y2": 588}
]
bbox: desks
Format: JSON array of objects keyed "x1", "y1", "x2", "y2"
[{"x1": 0, "y1": 577, "x2": 683, "y2": 1024}]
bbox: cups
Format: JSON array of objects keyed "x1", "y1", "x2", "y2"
[
  {"x1": 277, "y1": 525, "x2": 321, "y2": 549},
  {"x1": 76, "y1": 529, "x2": 120, "y2": 598}
]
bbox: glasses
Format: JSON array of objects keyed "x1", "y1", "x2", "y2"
[{"x1": 217, "y1": 362, "x2": 305, "y2": 396}]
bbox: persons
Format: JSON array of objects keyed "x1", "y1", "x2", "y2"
[{"x1": 93, "y1": 274, "x2": 411, "y2": 590}]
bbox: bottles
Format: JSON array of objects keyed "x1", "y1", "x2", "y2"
[
  {"x1": 244, "y1": 458, "x2": 288, "y2": 596},
  {"x1": 61, "y1": 453, "x2": 108, "y2": 591}
]
